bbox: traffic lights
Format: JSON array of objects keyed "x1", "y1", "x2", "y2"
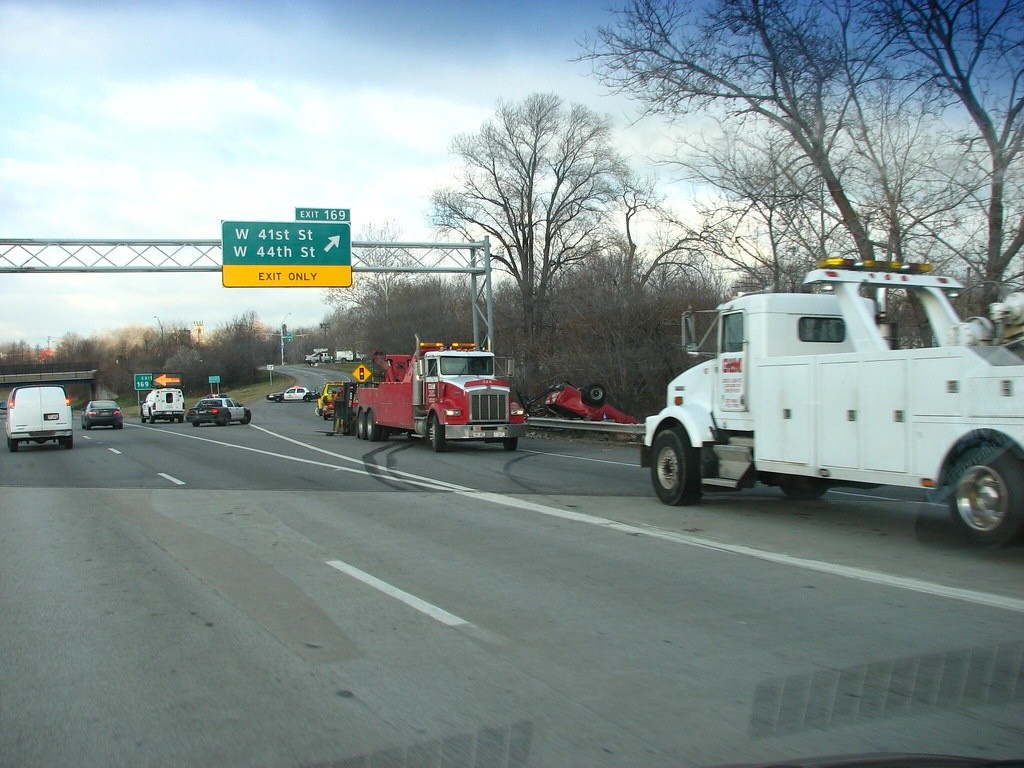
[{"x1": 358, "y1": 367, "x2": 367, "y2": 381}]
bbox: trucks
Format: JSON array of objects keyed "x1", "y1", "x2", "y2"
[
  {"x1": 303, "y1": 345, "x2": 370, "y2": 365},
  {"x1": 633, "y1": 256, "x2": 1024, "y2": 547}
]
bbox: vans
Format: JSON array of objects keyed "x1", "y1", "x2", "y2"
[
  {"x1": 139, "y1": 387, "x2": 185, "y2": 425},
  {"x1": 0, "y1": 383, "x2": 74, "y2": 454}
]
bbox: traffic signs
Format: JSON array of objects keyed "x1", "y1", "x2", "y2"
[{"x1": 222, "y1": 220, "x2": 353, "y2": 287}]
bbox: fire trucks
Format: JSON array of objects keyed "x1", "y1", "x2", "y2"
[{"x1": 333, "y1": 333, "x2": 528, "y2": 452}]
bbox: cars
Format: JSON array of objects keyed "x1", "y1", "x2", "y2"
[
  {"x1": 185, "y1": 395, "x2": 252, "y2": 427},
  {"x1": 544, "y1": 379, "x2": 639, "y2": 425},
  {"x1": 80, "y1": 400, "x2": 124, "y2": 430},
  {"x1": 317, "y1": 381, "x2": 348, "y2": 419},
  {"x1": 265, "y1": 386, "x2": 319, "y2": 403}
]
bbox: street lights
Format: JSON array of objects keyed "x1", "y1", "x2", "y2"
[
  {"x1": 279, "y1": 311, "x2": 293, "y2": 365},
  {"x1": 152, "y1": 315, "x2": 165, "y2": 367}
]
